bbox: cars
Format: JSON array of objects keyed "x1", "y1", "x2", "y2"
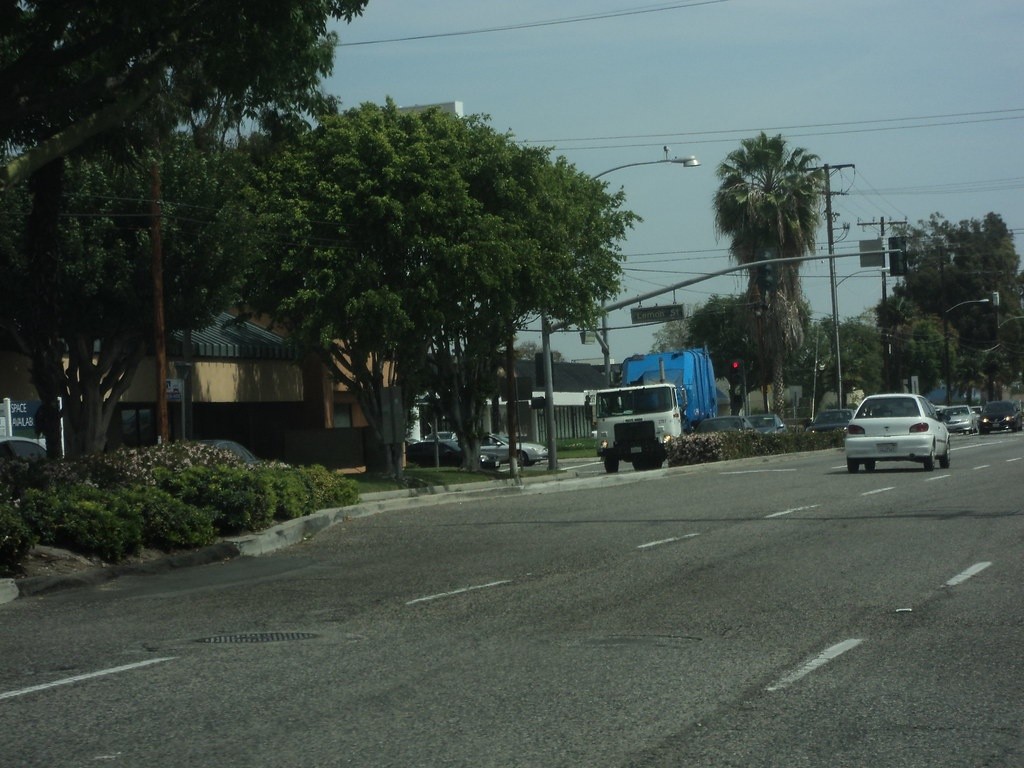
[
  {"x1": 978, "y1": 400, "x2": 1022, "y2": 434},
  {"x1": 692, "y1": 416, "x2": 761, "y2": 435},
  {"x1": 970, "y1": 406, "x2": 984, "y2": 417},
  {"x1": 480, "y1": 434, "x2": 548, "y2": 466},
  {"x1": 407, "y1": 441, "x2": 501, "y2": 470},
  {"x1": 928, "y1": 406, "x2": 947, "y2": 419},
  {"x1": 806, "y1": 409, "x2": 855, "y2": 432},
  {"x1": 0, "y1": 437, "x2": 47, "y2": 464},
  {"x1": 845, "y1": 393, "x2": 951, "y2": 472},
  {"x1": 196, "y1": 438, "x2": 266, "y2": 467},
  {"x1": 941, "y1": 405, "x2": 977, "y2": 434},
  {"x1": 747, "y1": 414, "x2": 788, "y2": 434},
  {"x1": 405, "y1": 437, "x2": 421, "y2": 448},
  {"x1": 425, "y1": 431, "x2": 458, "y2": 442}
]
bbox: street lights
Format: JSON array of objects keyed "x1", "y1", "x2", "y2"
[
  {"x1": 542, "y1": 156, "x2": 702, "y2": 471},
  {"x1": 833, "y1": 266, "x2": 891, "y2": 409},
  {"x1": 943, "y1": 299, "x2": 990, "y2": 405}
]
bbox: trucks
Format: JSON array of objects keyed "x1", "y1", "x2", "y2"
[{"x1": 584, "y1": 344, "x2": 718, "y2": 473}]
